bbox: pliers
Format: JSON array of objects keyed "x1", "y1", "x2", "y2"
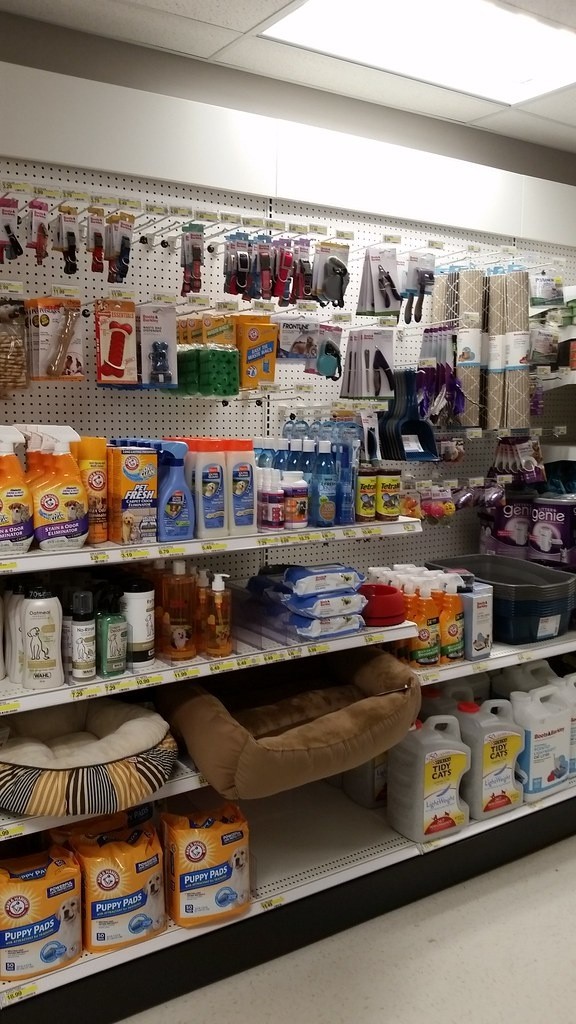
[{"x1": 378, "y1": 264, "x2": 403, "y2": 308}]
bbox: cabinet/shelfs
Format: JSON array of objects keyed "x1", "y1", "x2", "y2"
[{"x1": 0, "y1": 154, "x2": 576, "y2": 1024}]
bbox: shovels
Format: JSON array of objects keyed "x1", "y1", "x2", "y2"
[{"x1": 381, "y1": 369, "x2": 440, "y2": 462}]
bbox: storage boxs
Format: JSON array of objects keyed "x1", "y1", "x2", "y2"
[
  {"x1": 425, "y1": 554, "x2": 576, "y2": 645},
  {"x1": 107, "y1": 446, "x2": 158, "y2": 545},
  {"x1": 236, "y1": 314, "x2": 278, "y2": 387}
]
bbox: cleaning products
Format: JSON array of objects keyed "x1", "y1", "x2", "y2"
[{"x1": 0, "y1": 402, "x2": 495, "y2": 697}]
